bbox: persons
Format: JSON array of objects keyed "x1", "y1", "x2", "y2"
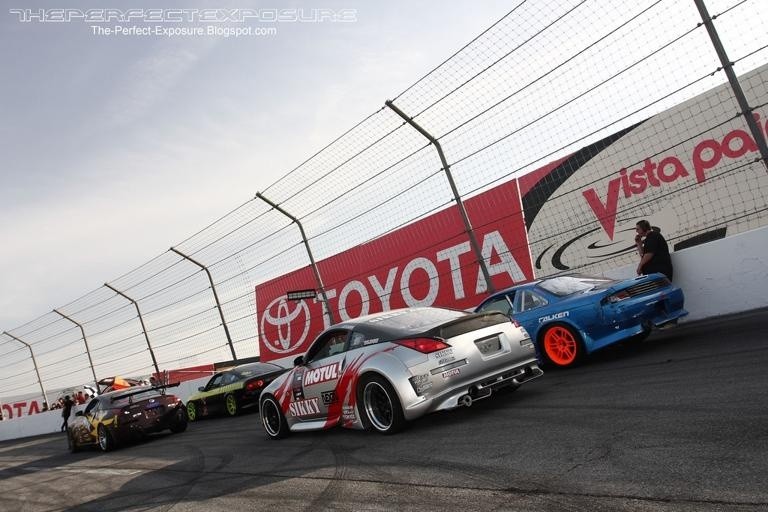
[
  {"x1": 42, "y1": 390, "x2": 93, "y2": 412},
  {"x1": 149, "y1": 376, "x2": 159, "y2": 384},
  {"x1": 634, "y1": 220, "x2": 678, "y2": 331},
  {"x1": 60, "y1": 395, "x2": 74, "y2": 432},
  {"x1": 142, "y1": 379, "x2": 152, "y2": 386},
  {"x1": 136, "y1": 379, "x2": 145, "y2": 386}
]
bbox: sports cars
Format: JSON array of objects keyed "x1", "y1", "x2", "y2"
[{"x1": 66, "y1": 270, "x2": 690, "y2": 452}]
